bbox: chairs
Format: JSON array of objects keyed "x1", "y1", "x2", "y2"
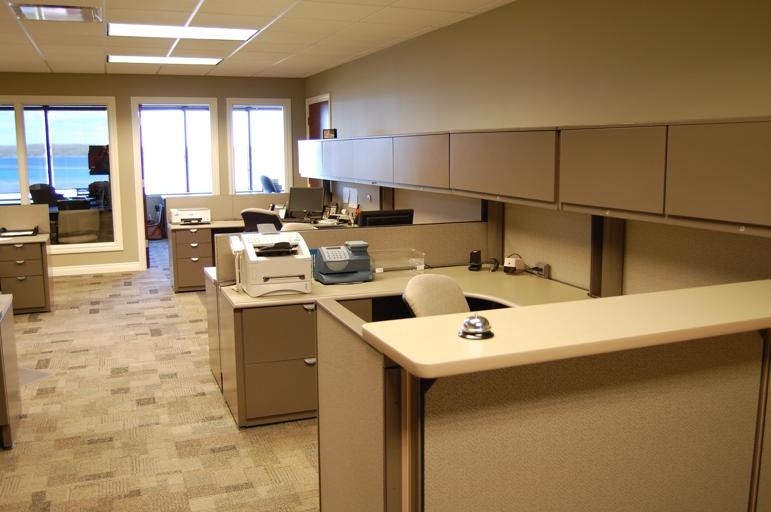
[
  {"x1": 402, "y1": 270, "x2": 473, "y2": 317},
  {"x1": 30, "y1": 184, "x2": 60, "y2": 204},
  {"x1": 241, "y1": 207, "x2": 284, "y2": 232}
]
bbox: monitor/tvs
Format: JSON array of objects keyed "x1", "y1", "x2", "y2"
[
  {"x1": 288, "y1": 187, "x2": 325, "y2": 220},
  {"x1": 359, "y1": 209, "x2": 413, "y2": 226}
]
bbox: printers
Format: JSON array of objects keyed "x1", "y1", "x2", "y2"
[
  {"x1": 169, "y1": 207, "x2": 211, "y2": 225},
  {"x1": 241, "y1": 231, "x2": 311, "y2": 297}
]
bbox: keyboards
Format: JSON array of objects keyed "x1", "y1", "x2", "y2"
[{"x1": 283, "y1": 218, "x2": 311, "y2": 223}]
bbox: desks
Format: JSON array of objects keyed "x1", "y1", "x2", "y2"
[
  {"x1": 313, "y1": 278, "x2": 771, "y2": 509},
  {"x1": 167, "y1": 220, "x2": 311, "y2": 293}
]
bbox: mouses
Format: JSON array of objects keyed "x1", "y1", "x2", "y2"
[{"x1": 312, "y1": 220, "x2": 317, "y2": 224}]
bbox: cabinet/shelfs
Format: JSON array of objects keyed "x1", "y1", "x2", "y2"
[
  {"x1": 202, "y1": 261, "x2": 374, "y2": 433},
  {"x1": 2, "y1": 232, "x2": 51, "y2": 314},
  {"x1": 297, "y1": 117, "x2": 771, "y2": 239}
]
